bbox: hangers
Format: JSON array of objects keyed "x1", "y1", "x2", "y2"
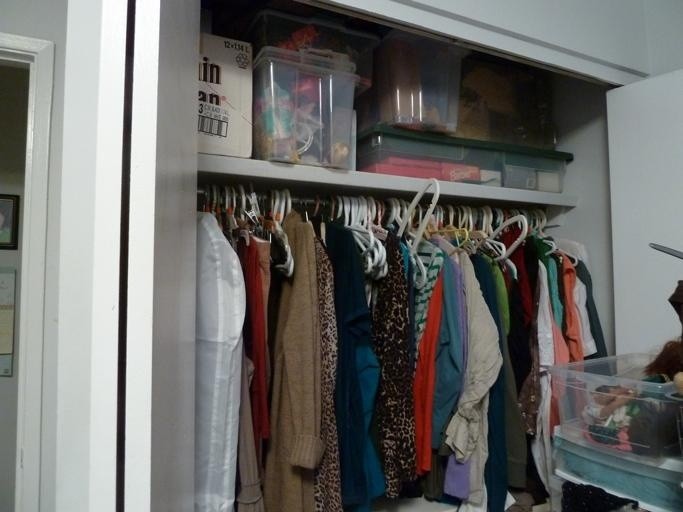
[{"x1": 196, "y1": 177, "x2": 585, "y2": 308}]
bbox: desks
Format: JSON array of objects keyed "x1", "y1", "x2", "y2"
[{"x1": 544, "y1": 415, "x2": 683, "y2": 512}]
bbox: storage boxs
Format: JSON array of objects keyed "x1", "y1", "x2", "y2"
[
  {"x1": 192, "y1": 12, "x2": 577, "y2": 193},
  {"x1": 542, "y1": 348, "x2": 681, "y2": 460}
]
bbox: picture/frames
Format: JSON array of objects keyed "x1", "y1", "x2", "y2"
[{"x1": 0, "y1": 193, "x2": 20, "y2": 254}]
